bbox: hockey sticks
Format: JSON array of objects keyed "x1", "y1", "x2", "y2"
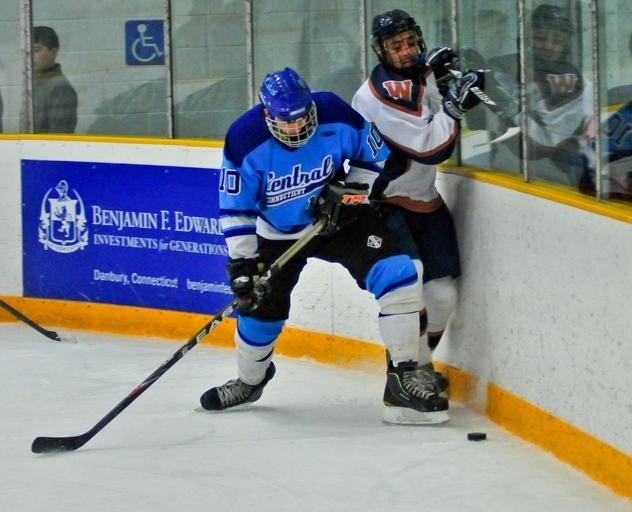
[
  {"x1": 0, "y1": 300, "x2": 105, "y2": 346},
  {"x1": 32, "y1": 215, "x2": 327, "y2": 454},
  {"x1": 444, "y1": 60, "x2": 521, "y2": 149}
]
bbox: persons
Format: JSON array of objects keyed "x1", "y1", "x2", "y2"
[
  {"x1": 194, "y1": 68, "x2": 453, "y2": 414},
  {"x1": 18, "y1": 26, "x2": 77, "y2": 133},
  {"x1": 461, "y1": 4, "x2": 595, "y2": 189},
  {"x1": 351, "y1": 9, "x2": 486, "y2": 401},
  {"x1": 579, "y1": 34, "x2": 631, "y2": 203}
]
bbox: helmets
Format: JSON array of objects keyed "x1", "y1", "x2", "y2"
[
  {"x1": 371, "y1": 8, "x2": 427, "y2": 70},
  {"x1": 425, "y1": 46, "x2": 458, "y2": 71},
  {"x1": 226, "y1": 254, "x2": 271, "y2": 315},
  {"x1": 311, "y1": 179, "x2": 369, "y2": 236},
  {"x1": 260, "y1": 67, "x2": 318, "y2": 149},
  {"x1": 531, "y1": 4, "x2": 573, "y2": 34}
]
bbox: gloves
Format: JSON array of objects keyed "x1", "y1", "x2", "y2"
[
  {"x1": 459, "y1": 47, "x2": 488, "y2": 70},
  {"x1": 485, "y1": 66, "x2": 520, "y2": 119},
  {"x1": 442, "y1": 69, "x2": 485, "y2": 121}
]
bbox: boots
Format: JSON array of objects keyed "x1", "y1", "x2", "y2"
[
  {"x1": 383, "y1": 351, "x2": 449, "y2": 412},
  {"x1": 201, "y1": 361, "x2": 275, "y2": 410}
]
